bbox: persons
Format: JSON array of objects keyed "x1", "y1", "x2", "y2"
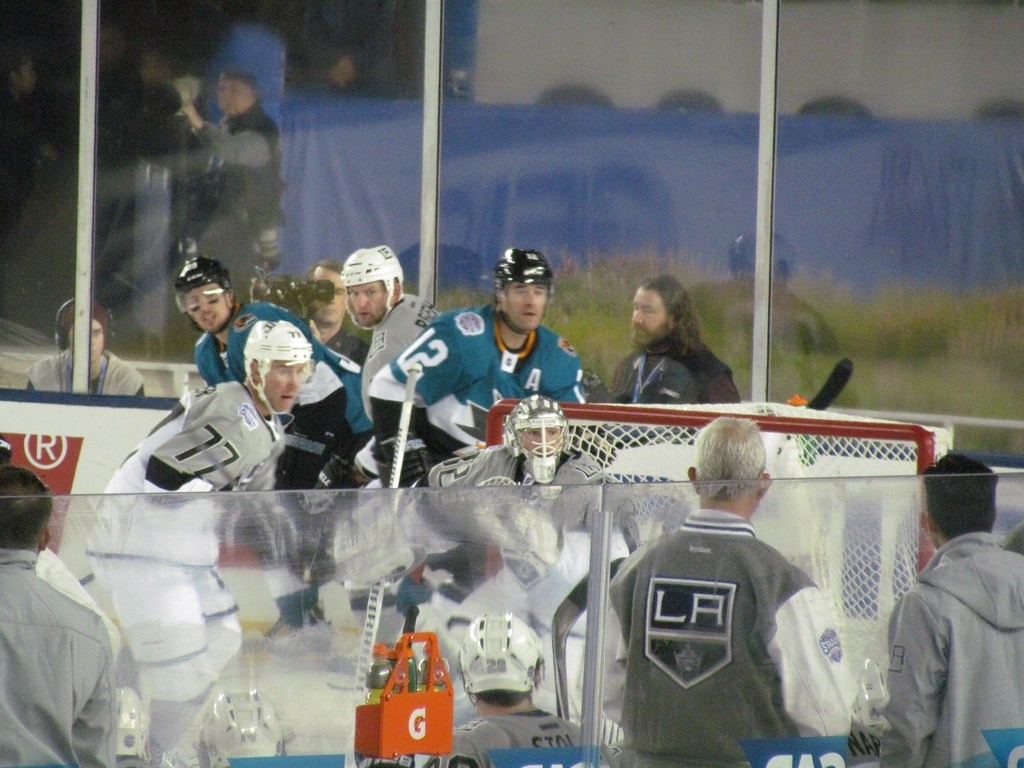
[
  {"x1": 582, "y1": 270, "x2": 741, "y2": 405},
  {"x1": 608, "y1": 416, "x2": 857, "y2": 768},
  {"x1": 661, "y1": 228, "x2": 854, "y2": 411},
  {"x1": 175, "y1": 246, "x2": 623, "y2": 691},
  {"x1": 879, "y1": 451, "x2": 1024, "y2": 768},
  {"x1": 0, "y1": 7, "x2": 279, "y2": 364},
  {"x1": 0, "y1": 436, "x2": 173, "y2": 768},
  {"x1": 83, "y1": 320, "x2": 316, "y2": 768},
  {"x1": 195, "y1": 679, "x2": 293, "y2": 768},
  {"x1": 25, "y1": 298, "x2": 146, "y2": 397},
  {"x1": 352, "y1": 611, "x2": 583, "y2": 768}
]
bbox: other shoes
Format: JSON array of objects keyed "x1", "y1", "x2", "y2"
[{"x1": 431, "y1": 580, "x2": 472, "y2": 651}]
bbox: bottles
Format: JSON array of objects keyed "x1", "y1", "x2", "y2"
[
  {"x1": 364, "y1": 649, "x2": 450, "y2": 704},
  {"x1": 786, "y1": 395, "x2": 816, "y2": 465}
]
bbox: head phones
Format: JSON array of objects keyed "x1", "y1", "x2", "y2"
[{"x1": 55, "y1": 297, "x2": 115, "y2": 350}]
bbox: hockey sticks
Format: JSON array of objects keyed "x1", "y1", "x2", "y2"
[
  {"x1": 354, "y1": 360, "x2": 424, "y2": 702},
  {"x1": 548, "y1": 555, "x2": 627, "y2": 722},
  {"x1": 181, "y1": 392, "x2": 278, "y2": 522},
  {"x1": 306, "y1": 518, "x2": 330, "y2": 577},
  {"x1": 810, "y1": 355, "x2": 854, "y2": 409}
]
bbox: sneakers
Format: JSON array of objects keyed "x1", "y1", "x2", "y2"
[{"x1": 262, "y1": 589, "x2": 332, "y2": 655}]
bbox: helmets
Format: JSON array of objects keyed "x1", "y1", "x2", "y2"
[
  {"x1": 243, "y1": 320, "x2": 313, "y2": 376},
  {"x1": 174, "y1": 256, "x2": 233, "y2": 293},
  {"x1": 201, "y1": 689, "x2": 281, "y2": 768},
  {"x1": 458, "y1": 611, "x2": 545, "y2": 694},
  {"x1": 114, "y1": 689, "x2": 151, "y2": 759},
  {"x1": 341, "y1": 245, "x2": 404, "y2": 292},
  {"x1": 494, "y1": 247, "x2": 553, "y2": 284},
  {"x1": 729, "y1": 232, "x2": 796, "y2": 284},
  {"x1": 503, "y1": 394, "x2": 569, "y2": 483}
]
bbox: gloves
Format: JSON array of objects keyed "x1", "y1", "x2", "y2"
[{"x1": 299, "y1": 459, "x2": 356, "y2": 514}]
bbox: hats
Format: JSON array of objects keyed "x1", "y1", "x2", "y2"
[{"x1": 62, "y1": 300, "x2": 108, "y2": 349}]
